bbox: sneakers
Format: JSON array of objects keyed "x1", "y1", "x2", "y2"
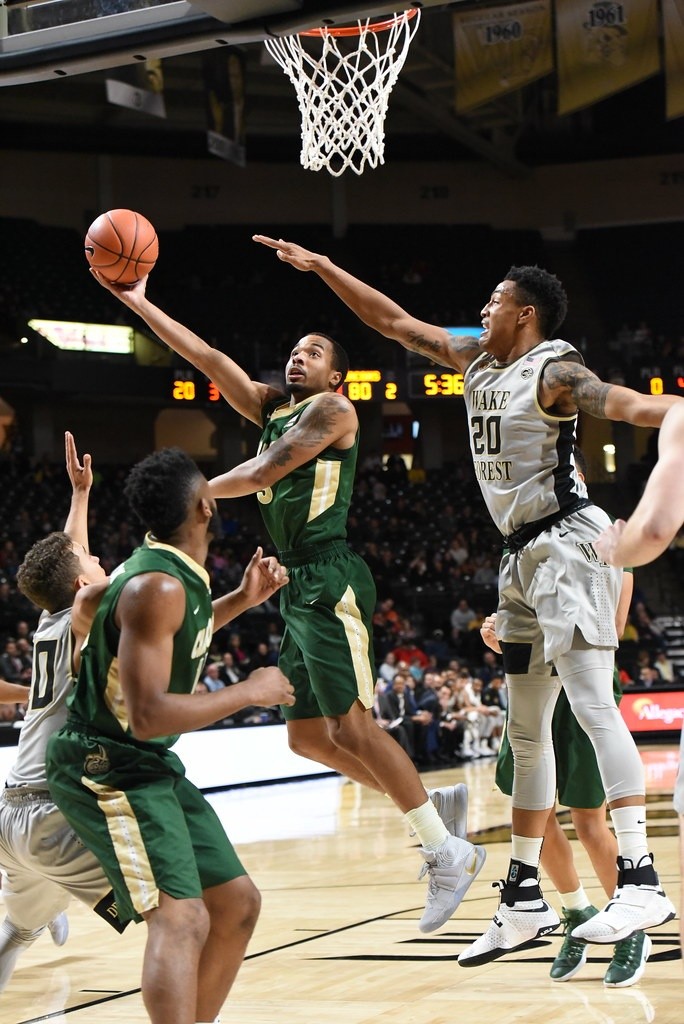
[
  {"x1": 570, "y1": 854, "x2": 676, "y2": 944},
  {"x1": 418, "y1": 840, "x2": 487, "y2": 936},
  {"x1": 458, "y1": 880, "x2": 561, "y2": 966},
  {"x1": 430, "y1": 783, "x2": 468, "y2": 839},
  {"x1": 550, "y1": 905, "x2": 598, "y2": 982},
  {"x1": 603, "y1": 932, "x2": 652, "y2": 988}
]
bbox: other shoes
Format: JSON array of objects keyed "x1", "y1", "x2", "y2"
[
  {"x1": 50, "y1": 914, "x2": 67, "y2": 943},
  {"x1": 461, "y1": 742, "x2": 498, "y2": 758}
]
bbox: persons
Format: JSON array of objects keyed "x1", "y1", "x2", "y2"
[
  {"x1": 616, "y1": 320, "x2": 651, "y2": 349},
  {"x1": 0, "y1": 678, "x2": 31, "y2": 704},
  {"x1": 594, "y1": 398, "x2": 684, "y2": 568},
  {"x1": 0, "y1": 422, "x2": 676, "y2": 763},
  {"x1": 0, "y1": 429, "x2": 136, "y2": 985},
  {"x1": 46, "y1": 451, "x2": 296, "y2": 1024},
  {"x1": 252, "y1": 233, "x2": 678, "y2": 967},
  {"x1": 85, "y1": 266, "x2": 485, "y2": 931},
  {"x1": 479, "y1": 567, "x2": 651, "y2": 989}
]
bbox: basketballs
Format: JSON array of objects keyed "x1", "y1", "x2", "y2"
[{"x1": 84, "y1": 207, "x2": 161, "y2": 284}]
